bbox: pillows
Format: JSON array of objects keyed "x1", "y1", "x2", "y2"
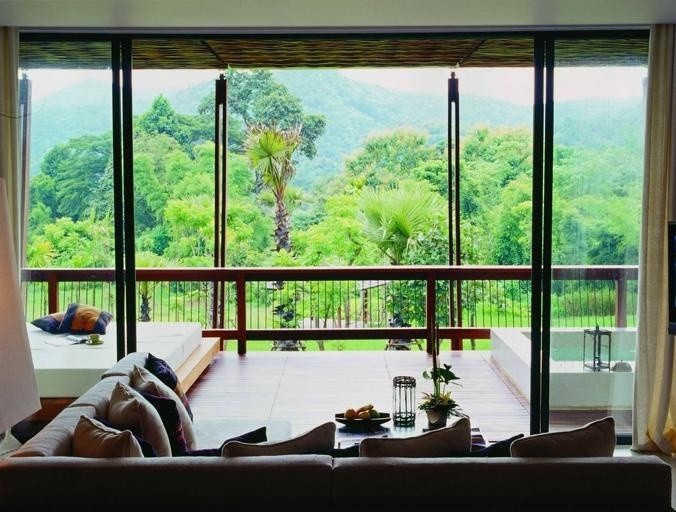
[
  {"x1": 510, "y1": 417, "x2": 617, "y2": 457},
  {"x1": 30, "y1": 312, "x2": 65, "y2": 332},
  {"x1": 222, "y1": 422, "x2": 335, "y2": 457},
  {"x1": 185, "y1": 427, "x2": 267, "y2": 456},
  {"x1": 335, "y1": 435, "x2": 388, "y2": 458},
  {"x1": 471, "y1": 433, "x2": 524, "y2": 457},
  {"x1": 58, "y1": 304, "x2": 113, "y2": 334},
  {"x1": 359, "y1": 418, "x2": 471, "y2": 458},
  {"x1": 74, "y1": 353, "x2": 199, "y2": 458}
]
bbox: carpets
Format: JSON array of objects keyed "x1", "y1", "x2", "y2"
[{"x1": 470, "y1": 429, "x2": 487, "y2": 444}]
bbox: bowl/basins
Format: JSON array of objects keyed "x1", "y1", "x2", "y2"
[
  {"x1": 339, "y1": 428, "x2": 390, "y2": 435},
  {"x1": 337, "y1": 409, "x2": 392, "y2": 427}
]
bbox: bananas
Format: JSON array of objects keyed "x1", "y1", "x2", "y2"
[{"x1": 355, "y1": 404, "x2": 374, "y2": 418}]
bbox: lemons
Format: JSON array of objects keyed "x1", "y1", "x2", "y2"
[{"x1": 368, "y1": 409, "x2": 379, "y2": 418}]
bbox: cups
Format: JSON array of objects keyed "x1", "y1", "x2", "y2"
[{"x1": 87, "y1": 333, "x2": 99, "y2": 343}]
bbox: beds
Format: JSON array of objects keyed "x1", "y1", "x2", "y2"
[{"x1": 21, "y1": 320, "x2": 220, "y2": 426}]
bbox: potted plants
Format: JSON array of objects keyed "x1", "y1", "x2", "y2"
[{"x1": 421, "y1": 363, "x2": 459, "y2": 431}]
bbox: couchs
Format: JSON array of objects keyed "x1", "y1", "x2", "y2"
[{"x1": 1, "y1": 352, "x2": 672, "y2": 511}]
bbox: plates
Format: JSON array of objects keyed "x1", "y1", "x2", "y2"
[{"x1": 85, "y1": 340, "x2": 104, "y2": 345}]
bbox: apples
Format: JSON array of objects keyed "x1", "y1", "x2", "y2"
[
  {"x1": 358, "y1": 412, "x2": 371, "y2": 419},
  {"x1": 345, "y1": 409, "x2": 357, "y2": 418}
]
